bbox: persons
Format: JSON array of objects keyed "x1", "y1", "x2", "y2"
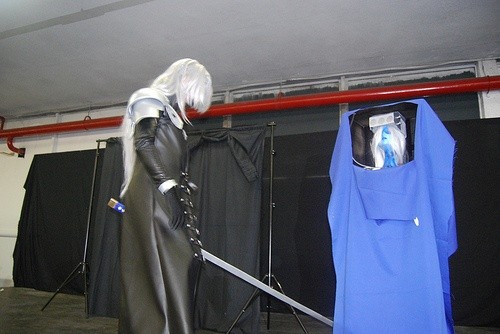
[
  {"x1": 356, "y1": 111, "x2": 410, "y2": 172},
  {"x1": 119, "y1": 57, "x2": 214, "y2": 334}
]
